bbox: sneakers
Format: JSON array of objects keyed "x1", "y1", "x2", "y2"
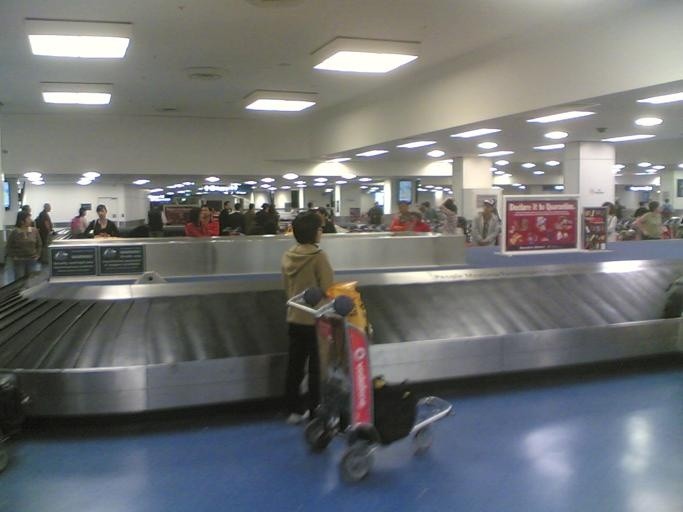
[{"x1": 287, "y1": 409, "x2": 311, "y2": 425}]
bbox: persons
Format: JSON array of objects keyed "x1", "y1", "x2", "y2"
[
  {"x1": 468, "y1": 198, "x2": 500, "y2": 248},
  {"x1": 2, "y1": 210, "x2": 43, "y2": 282},
  {"x1": 35, "y1": 203, "x2": 53, "y2": 265},
  {"x1": 147, "y1": 203, "x2": 167, "y2": 238},
  {"x1": 368, "y1": 202, "x2": 384, "y2": 224},
  {"x1": 318, "y1": 207, "x2": 337, "y2": 233},
  {"x1": 185, "y1": 200, "x2": 280, "y2": 238},
  {"x1": 279, "y1": 210, "x2": 340, "y2": 431},
  {"x1": 21, "y1": 204, "x2": 31, "y2": 214},
  {"x1": 600, "y1": 195, "x2": 674, "y2": 243},
  {"x1": 82, "y1": 204, "x2": 120, "y2": 239},
  {"x1": 390, "y1": 198, "x2": 457, "y2": 232},
  {"x1": 70, "y1": 208, "x2": 88, "y2": 239}
]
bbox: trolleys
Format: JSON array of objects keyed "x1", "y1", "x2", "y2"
[{"x1": 285, "y1": 286, "x2": 452, "y2": 485}]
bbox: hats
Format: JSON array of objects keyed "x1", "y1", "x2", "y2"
[{"x1": 483, "y1": 198, "x2": 496, "y2": 208}]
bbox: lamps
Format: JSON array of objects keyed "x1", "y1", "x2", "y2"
[
  {"x1": 40, "y1": 82, "x2": 113, "y2": 105},
  {"x1": 308, "y1": 38, "x2": 422, "y2": 74},
  {"x1": 26, "y1": 20, "x2": 132, "y2": 58},
  {"x1": 241, "y1": 90, "x2": 320, "y2": 111}
]
